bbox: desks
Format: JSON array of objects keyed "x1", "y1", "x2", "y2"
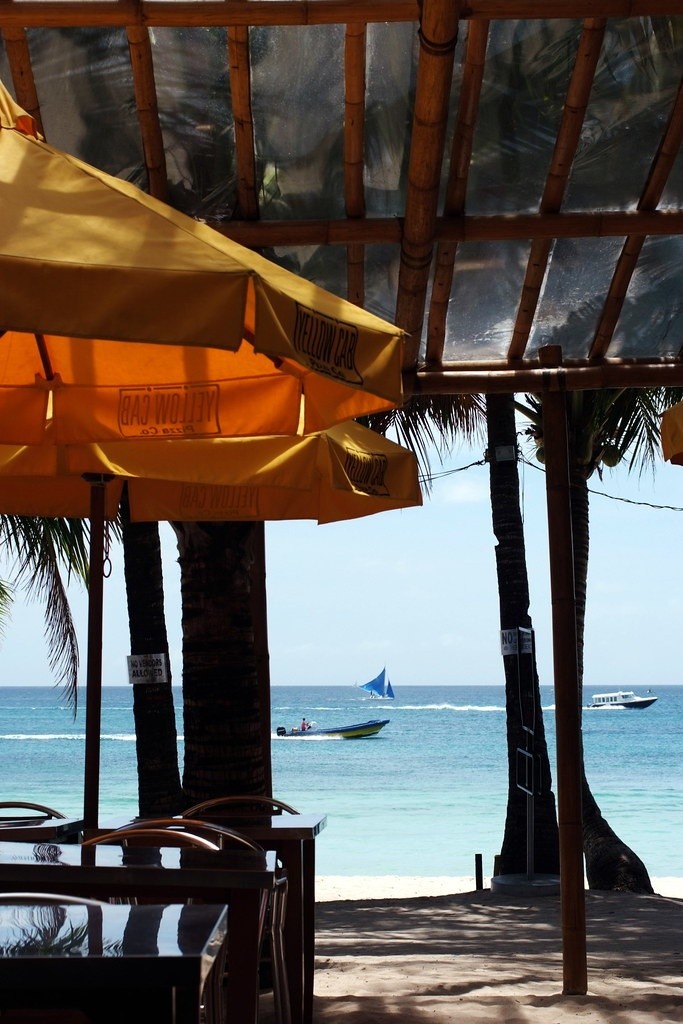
[
  {"x1": 0, "y1": 842, "x2": 278, "y2": 1024},
  {"x1": 81, "y1": 811, "x2": 328, "y2": 1024},
  {"x1": 0, "y1": 902, "x2": 228, "y2": 1024}
]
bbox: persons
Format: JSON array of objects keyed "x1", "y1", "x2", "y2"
[{"x1": 301, "y1": 717, "x2": 307, "y2": 731}]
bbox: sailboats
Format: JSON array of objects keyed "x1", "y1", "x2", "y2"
[{"x1": 358, "y1": 666, "x2": 395, "y2": 700}]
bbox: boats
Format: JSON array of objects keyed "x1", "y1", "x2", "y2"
[
  {"x1": 277, "y1": 720, "x2": 390, "y2": 738},
  {"x1": 587, "y1": 691, "x2": 658, "y2": 709}
]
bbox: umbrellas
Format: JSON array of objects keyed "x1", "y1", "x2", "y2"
[{"x1": 0, "y1": 84, "x2": 422, "y2": 845}]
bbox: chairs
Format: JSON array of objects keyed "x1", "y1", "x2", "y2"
[{"x1": 0, "y1": 794, "x2": 303, "y2": 1024}]
